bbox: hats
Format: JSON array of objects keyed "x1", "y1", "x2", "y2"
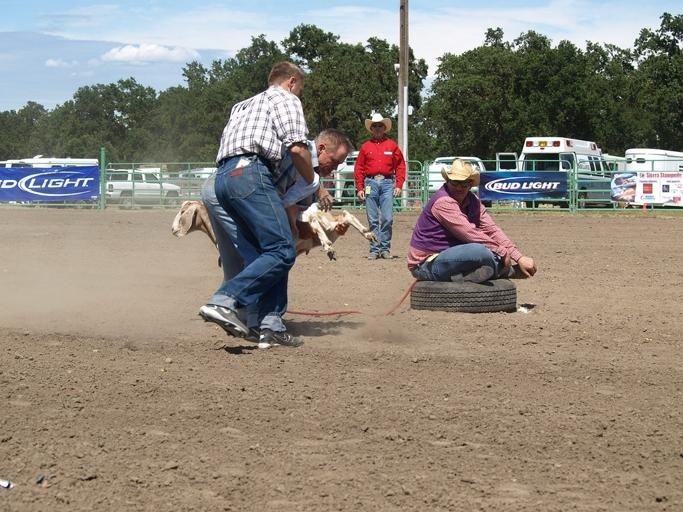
[
  {"x1": 364, "y1": 112, "x2": 393, "y2": 133},
  {"x1": 440, "y1": 158, "x2": 482, "y2": 188}
]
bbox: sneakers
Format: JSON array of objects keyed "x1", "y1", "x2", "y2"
[
  {"x1": 256, "y1": 328, "x2": 305, "y2": 349},
  {"x1": 451, "y1": 264, "x2": 496, "y2": 284},
  {"x1": 197, "y1": 303, "x2": 251, "y2": 340},
  {"x1": 511, "y1": 263, "x2": 529, "y2": 280},
  {"x1": 381, "y1": 251, "x2": 393, "y2": 260},
  {"x1": 367, "y1": 251, "x2": 380, "y2": 260}
]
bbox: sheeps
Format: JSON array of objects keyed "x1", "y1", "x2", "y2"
[{"x1": 170, "y1": 197, "x2": 378, "y2": 262}]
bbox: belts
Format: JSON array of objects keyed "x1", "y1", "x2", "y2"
[
  {"x1": 215, "y1": 157, "x2": 232, "y2": 168},
  {"x1": 369, "y1": 175, "x2": 392, "y2": 180}
]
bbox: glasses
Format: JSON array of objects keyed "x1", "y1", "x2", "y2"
[{"x1": 448, "y1": 179, "x2": 472, "y2": 188}]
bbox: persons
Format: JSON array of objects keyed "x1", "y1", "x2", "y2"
[
  {"x1": 200, "y1": 127, "x2": 353, "y2": 344},
  {"x1": 350, "y1": 106, "x2": 406, "y2": 260},
  {"x1": 403, "y1": 156, "x2": 537, "y2": 283},
  {"x1": 195, "y1": 61, "x2": 334, "y2": 350}
]
